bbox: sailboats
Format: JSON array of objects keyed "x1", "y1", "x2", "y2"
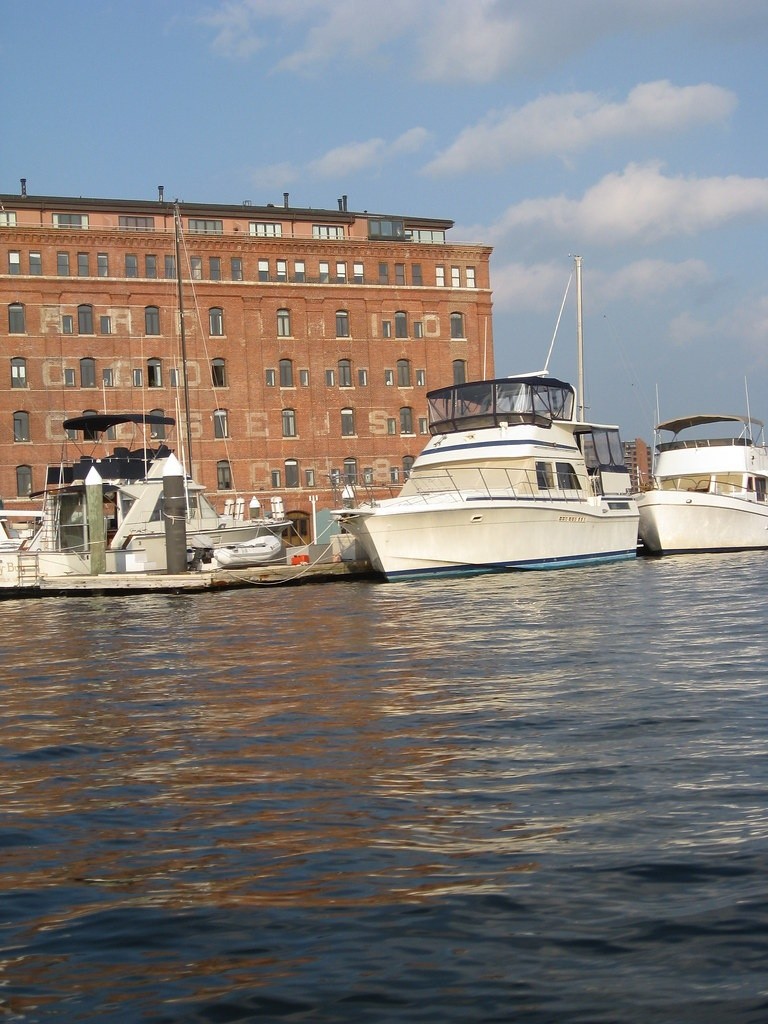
[
  {"x1": 329, "y1": 251, "x2": 641, "y2": 581},
  {"x1": 0, "y1": 203, "x2": 295, "y2": 579}
]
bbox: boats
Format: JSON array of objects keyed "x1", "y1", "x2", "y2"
[
  {"x1": 635, "y1": 382, "x2": 768, "y2": 554},
  {"x1": 185, "y1": 532, "x2": 282, "y2": 572}
]
